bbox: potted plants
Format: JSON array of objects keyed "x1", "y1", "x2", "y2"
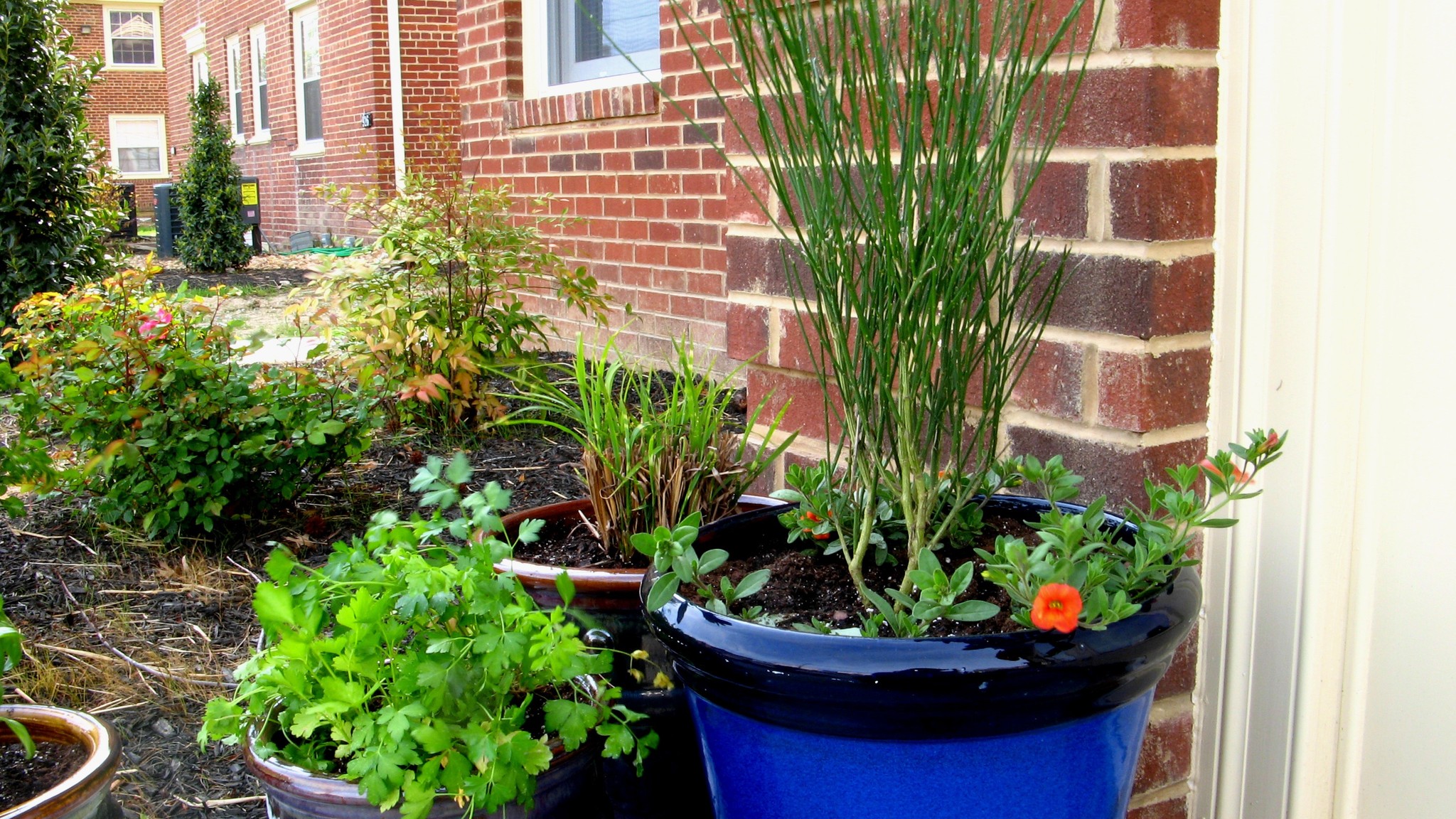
[
  {"x1": 197, "y1": 318, "x2": 790, "y2": 819},
  {"x1": 577, "y1": 1, "x2": 1286, "y2": 819},
  {"x1": 0, "y1": 594, "x2": 122, "y2": 819}
]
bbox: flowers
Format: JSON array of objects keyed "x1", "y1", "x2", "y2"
[{"x1": 630, "y1": 426, "x2": 1287, "y2": 633}]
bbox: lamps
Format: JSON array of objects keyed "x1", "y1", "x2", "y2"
[{"x1": 81, "y1": 26, "x2": 91, "y2": 34}]
